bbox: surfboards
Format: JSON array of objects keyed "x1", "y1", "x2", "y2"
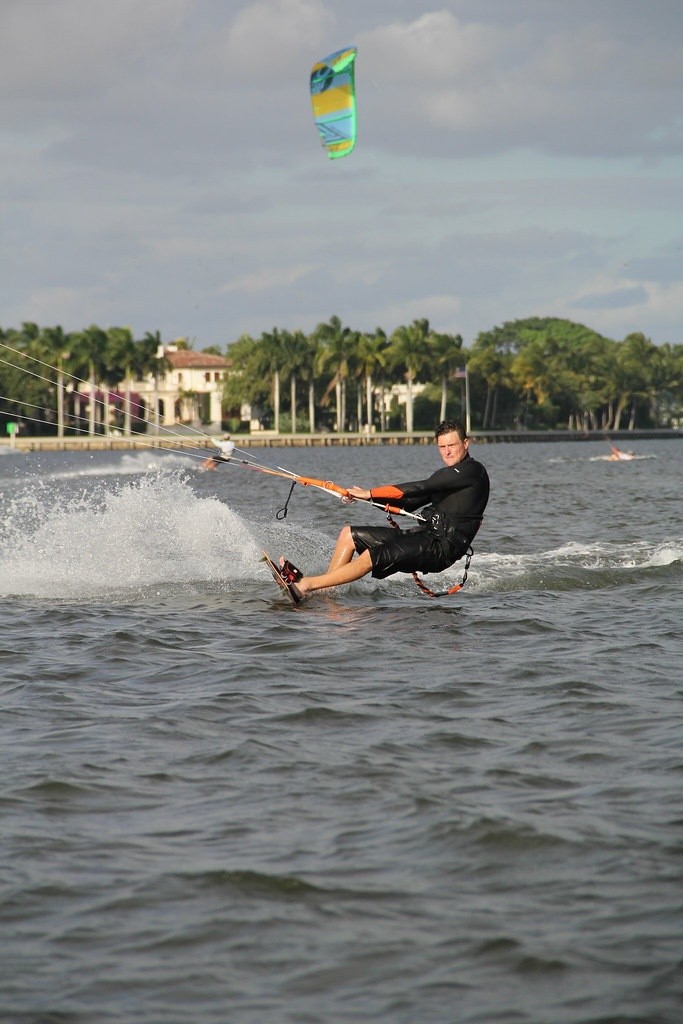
[{"x1": 260, "y1": 551, "x2": 297, "y2": 605}]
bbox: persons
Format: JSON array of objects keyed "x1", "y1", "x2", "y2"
[
  {"x1": 279, "y1": 419, "x2": 490, "y2": 598},
  {"x1": 610, "y1": 447, "x2": 634, "y2": 461},
  {"x1": 203, "y1": 434, "x2": 235, "y2": 469}
]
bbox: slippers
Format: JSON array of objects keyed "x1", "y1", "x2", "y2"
[{"x1": 279, "y1": 561, "x2": 303, "y2": 604}]
